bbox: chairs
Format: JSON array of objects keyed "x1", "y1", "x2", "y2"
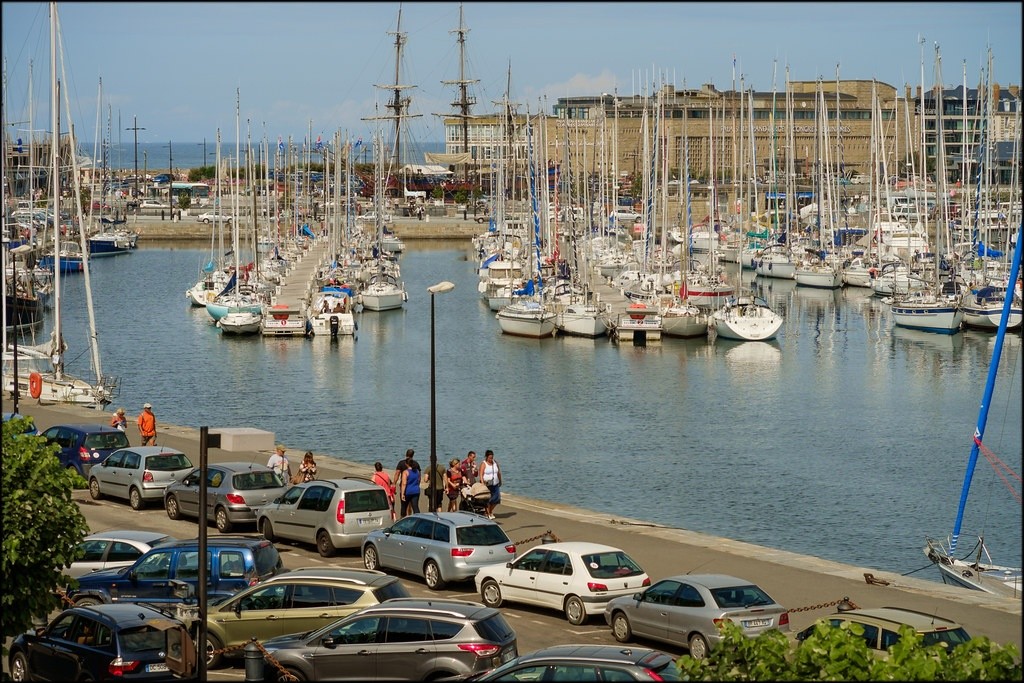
[
  {"x1": 549, "y1": 557, "x2": 565, "y2": 573},
  {"x1": 719, "y1": 597, "x2": 727, "y2": 607},
  {"x1": 78, "y1": 626, "x2": 95, "y2": 645},
  {"x1": 178, "y1": 554, "x2": 189, "y2": 578},
  {"x1": 742, "y1": 595, "x2": 755, "y2": 606}
]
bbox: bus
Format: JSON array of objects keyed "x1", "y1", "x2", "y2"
[{"x1": 161, "y1": 183, "x2": 211, "y2": 207}]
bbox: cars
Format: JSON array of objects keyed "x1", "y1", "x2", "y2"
[
  {"x1": 549, "y1": 201, "x2": 570, "y2": 210},
  {"x1": 2, "y1": 414, "x2": 795, "y2": 660},
  {"x1": 197, "y1": 211, "x2": 241, "y2": 224},
  {"x1": 621, "y1": 197, "x2": 642, "y2": 205},
  {"x1": 311, "y1": 173, "x2": 333, "y2": 182},
  {"x1": 474, "y1": 212, "x2": 512, "y2": 224},
  {"x1": 268, "y1": 170, "x2": 285, "y2": 182},
  {"x1": 244, "y1": 185, "x2": 272, "y2": 196},
  {"x1": 87, "y1": 173, "x2": 176, "y2": 209}
]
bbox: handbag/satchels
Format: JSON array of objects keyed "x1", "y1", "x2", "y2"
[
  {"x1": 390, "y1": 485, "x2": 397, "y2": 494},
  {"x1": 485, "y1": 480, "x2": 493, "y2": 486},
  {"x1": 117, "y1": 424, "x2": 125, "y2": 433},
  {"x1": 293, "y1": 463, "x2": 306, "y2": 484},
  {"x1": 425, "y1": 488, "x2": 431, "y2": 495}
]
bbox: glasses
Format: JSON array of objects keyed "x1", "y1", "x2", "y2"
[{"x1": 147, "y1": 407, "x2": 152, "y2": 409}]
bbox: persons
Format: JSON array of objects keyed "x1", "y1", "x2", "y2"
[
  {"x1": 372, "y1": 449, "x2": 502, "y2": 520},
  {"x1": 113, "y1": 208, "x2": 137, "y2": 221},
  {"x1": 138, "y1": 403, "x2": 157, "y2": 446},
  {"x1": 324, "y1": 300, "x2": 345, "y2": 313},
  {"x1": 517, "y1": 277, "x2": 539, "y2": 289},
  {"x1": 172, "y1": 206, "x2": 175, "y2": 218},
  {"x1": 366, "y1": 203, "x2": 368, "y2": 213},
  {"x1": 111, "y1": 408, "x2": 128, "y2": 435},
  {"x1": 314, "y1": 204, "x2": 317, "y2": 220},
  {"x1": 267, "y1": 445, "x2": 292, "y2": 486},
  {"x1": 298, "y1": 452, "x2": 316, "y2": 482},
  {"x1": 1017, "y1": 277, "x2": 1023, "y2": 286},
  {"x1": 358, "y1": 204, "x2": 361, "y2": 215},
  {"x1": 408, "y1": 202, "x2": 425, "y2": 220}
]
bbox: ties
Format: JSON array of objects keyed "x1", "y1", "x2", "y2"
[{"x1": 281, "y1": 456, "x2": 284, "y2": 473}]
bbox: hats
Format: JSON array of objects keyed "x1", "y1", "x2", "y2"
[
  {"x1": 144, "y1": 402, "x2": 152, "y2": 408},
  {"x1": 276, "y1": 445, "x2": 287, "y2": 451}
]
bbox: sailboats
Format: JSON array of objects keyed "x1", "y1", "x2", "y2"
[
  {"x1": 891, "y1": 326, "x2": 964, "y2": 352},
  {"x1": 185, "y1": 2, "x2": 481, "y2": 338},
  {"x1": 924, "y1": 220, "x2": 1022, "y2": 597},
  {"x1": 471, "y1": 34, "x2": 1023, "y2": 341},
  {"x1": 2, "y1": 3, "x2": 134, "y2": 410}
]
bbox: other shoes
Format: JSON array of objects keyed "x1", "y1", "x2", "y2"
[{"x1": 488, "y1": 514, "x2": 495, "y2": 520}]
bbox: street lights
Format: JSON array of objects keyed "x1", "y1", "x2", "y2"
[{"x1": 427, "y1": 281, "x2": 455, "y2": 513}]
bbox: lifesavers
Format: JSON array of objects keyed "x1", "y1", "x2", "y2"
[
  {"x1": 136, "y1": 228, "x2": 141, "y2": 234},
  {"x1": 721, "y1": 234, "x2": 725, "y2": 241},
  {"x1": 513, "y1": 241, "x2": 520, "y2": 248},
  {"x1": 207, "y1": 293, "x2": 215, "y2": 301}
]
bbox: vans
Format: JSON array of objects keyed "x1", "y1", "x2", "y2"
[{"x1": 851, "y1": 175, "x2": 871, "y2": 185}]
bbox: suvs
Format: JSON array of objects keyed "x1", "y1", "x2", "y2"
[
  {"x1": 8, "y1": 531, "x2": 681, "y2": 683},
  {"x1": 793, "y1": 607, "x2": 973, "y2": 677},
  {"x1": 610, "y1": 209, "x2": 642, "y2": 223},
  {"x1": 550, "y1": 208, "x2": 583, "y2": 222}
]
